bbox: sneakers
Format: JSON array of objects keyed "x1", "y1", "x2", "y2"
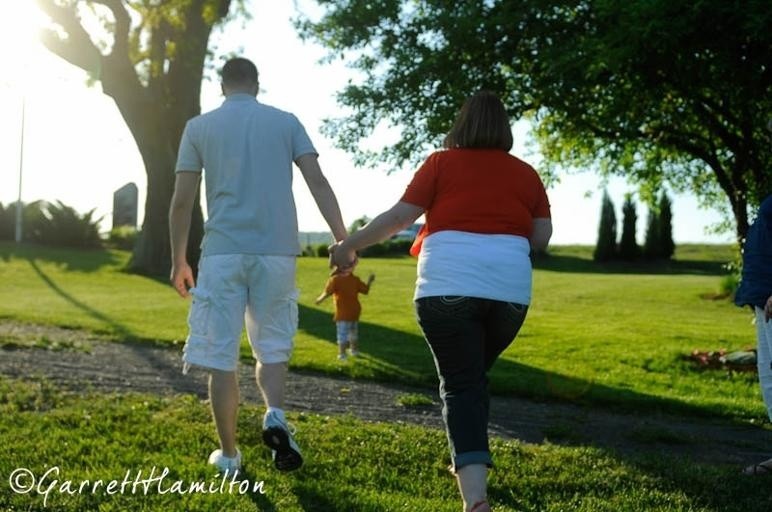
[
  {"x1": 262, "y1": 410, "x2": 303, "y2": 472},
  {"x1": 207, "y1": 448, "x2": 243, "y2": 475}
]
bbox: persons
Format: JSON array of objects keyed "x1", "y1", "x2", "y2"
[
  {"x1": 169, "y1": 56, "x2": 358, "y2": 483},
  {"x1": 732, "y1": 191, "x2": 772, "y2": 478},
  {"x1": 328, "y1": 91, "x2": 553, "y2": 511},
  {"x1": 314, "y1": 265, "x2": 376, "y2": 360}
]
